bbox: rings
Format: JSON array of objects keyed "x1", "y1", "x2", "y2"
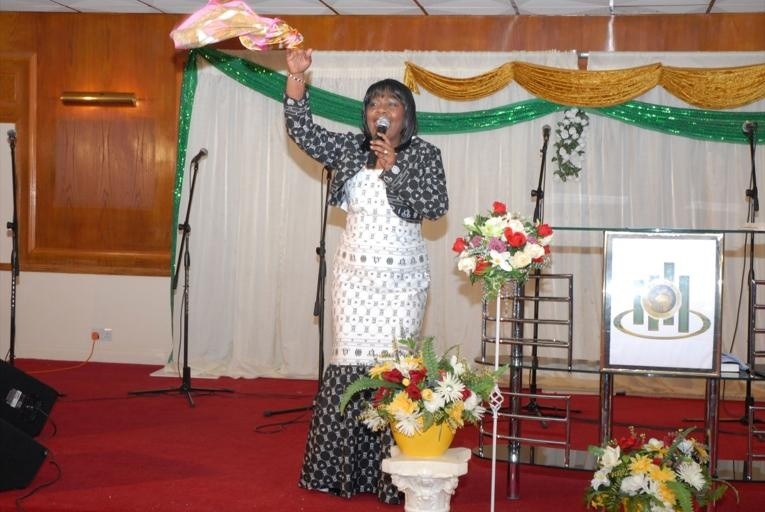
[{"x1": 384, "y1": 148, "x2": 388, "y2": 155}]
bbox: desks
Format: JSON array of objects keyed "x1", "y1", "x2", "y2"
[{"x1": 473, "y1": 353, "x2": 765, "y2": 501}]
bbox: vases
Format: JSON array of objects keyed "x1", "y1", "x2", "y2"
[{"x1": 387, "y1": 402, "x2": 464, "y2": 462}]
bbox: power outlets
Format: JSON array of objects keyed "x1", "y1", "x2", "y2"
[{"x1": 88, "y1": 328, "x2": 112, "y2": 341}]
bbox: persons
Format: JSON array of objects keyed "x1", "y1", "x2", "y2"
[{"x1": 284, "y1": 46, "x2": 449, "y2": 505}]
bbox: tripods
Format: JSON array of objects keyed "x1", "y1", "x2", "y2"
[
  {"x1": 129, "y1": 166, "x2": 234, "y2": 404},
  {"x1": 681, "y1": 150, "x2": 765, "y2": 441},
  {"x1": 472, "y1": 153, "x2": 582, "y2": 428}
]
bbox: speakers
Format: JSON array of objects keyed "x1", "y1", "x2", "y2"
[
  {"x1": 0, "y1": 415, "x2": 49, "y2": 494},
  {"x1": 0, "y1": 359, "x2": 61, "y2": 436}
]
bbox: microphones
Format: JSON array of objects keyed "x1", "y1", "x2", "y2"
[
  {"x1": 366, "y1": 116, "x2": 390, "y2": 167},
  {"x1": 191, "y1": 147, "x2": 210, "y2": 163},
  {"x1": 741, "y1": 119, "x2": 755, "y2": 138},
  {"x1": 7, "y1": 128, "x2": 18, "y2": 147},
  {"x1": 542, "y1": 123, "x2": 556, "y2": 142},
  {"x1": 326, "y1": 157, "x2": 341, "y2": 171}
]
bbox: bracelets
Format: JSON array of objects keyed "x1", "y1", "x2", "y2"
[{"x1": 287, "y1": 72, "x2": 306, "y2": 84}]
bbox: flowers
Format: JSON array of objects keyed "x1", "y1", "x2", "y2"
[
  {"x1": 583, "y1": 422, "x2": 739, "y2": 512},
  {"x1": 451, "y1": 200, "x2": 555, "y2": 303},
  {"x1": 340, "y1": 325, "x2": 511, "y2": 438}
]
